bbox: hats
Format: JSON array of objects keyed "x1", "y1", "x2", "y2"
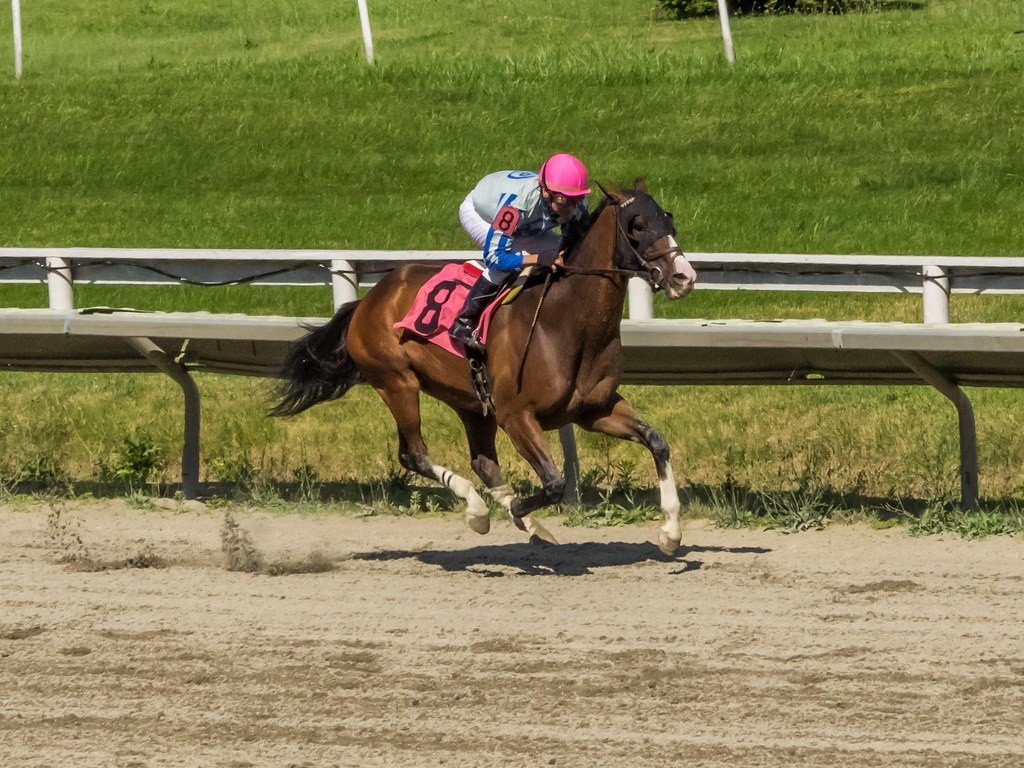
[{"x1": 536, "y1": 153, "x2": 592, "y2": 196}]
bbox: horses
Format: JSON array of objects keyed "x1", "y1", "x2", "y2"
[{"x1": 253, "y1": 171, "x2": 698, "y2": 557}]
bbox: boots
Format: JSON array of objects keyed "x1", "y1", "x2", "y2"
[{"x1": 447, "y1": 274, "x2": 500, "y2": 353}]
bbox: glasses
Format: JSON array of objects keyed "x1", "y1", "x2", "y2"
[{"x1": 554, "y1": 193, "x2": 585, "y2": 206}]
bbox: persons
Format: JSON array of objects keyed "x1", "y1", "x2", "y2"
[{"x1": 448, "y1": 154, "x2": 591, "y2": 348}]
